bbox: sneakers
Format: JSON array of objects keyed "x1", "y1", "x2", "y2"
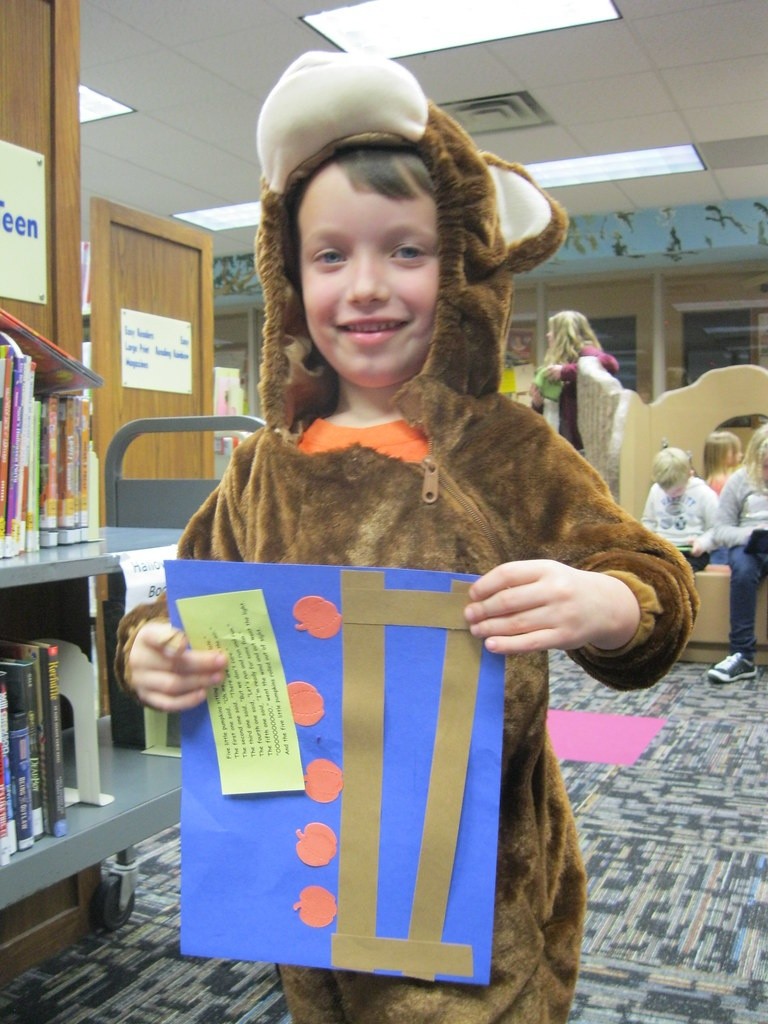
[{"x1": 708, "y1": 652, "x2": 757, "y2": 683}]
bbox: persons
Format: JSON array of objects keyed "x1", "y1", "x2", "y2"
[
  {"x1": 706, "y1": 424, "x2": 768, "y2": 682},
  {"x1": 703, "y1": 432, "x2": 742, "y2": 565},
  {"x1": 111, "y1": 48, "x2": 704, "y2": 1024},
  {"x1": 529, "y1": 309, "x2": 619, "y2": 458},
  {"x1": 638, "y1": 444, "x2": 719, "y2": 574}
]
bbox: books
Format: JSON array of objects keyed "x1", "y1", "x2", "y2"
[
  {"x1": 0, "y1": 634, "x2": 69, "y2": 866},
  {"x1": 0, "y1": 308, "x2": 103, "y2": 560}
]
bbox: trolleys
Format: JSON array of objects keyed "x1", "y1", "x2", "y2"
[{"x1": 1, "y1": 415, "x2": 271, "y2": 925}]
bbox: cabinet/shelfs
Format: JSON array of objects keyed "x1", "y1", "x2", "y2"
[{"x1": 0, "y1": 302, "x2": 274, "y2": 933}]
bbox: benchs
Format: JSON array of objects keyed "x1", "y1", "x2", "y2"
[{"x1": 620, "y1": 363, "x2": 768, "y2": 667}]
